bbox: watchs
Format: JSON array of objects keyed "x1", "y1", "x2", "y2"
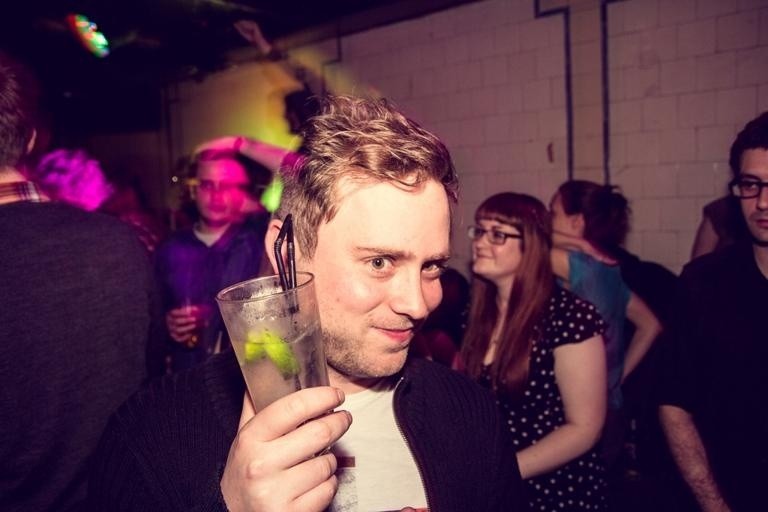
[{"x1": 233, "y1": 135, "x2": 244, "y2": 154}]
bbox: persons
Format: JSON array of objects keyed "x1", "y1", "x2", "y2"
[
  {"x1": 691, "y1": 192, "x2": 745, "y2": 258},
  {"x1": 549, "y1": 180, "x2": 663, "y2": 512},
  {"x1": 650, "y1": 107, "x2": 768, "y2": 512},
  {"x1": 101, "y1": 168, "x2": 165, "y2": 254},
  {"x1": 150, "y1": 136, "x2": 305, "y2": 411},
  {"x1": 0, "y1": 50, "x2": 164, "y2": 512},
  {"x1": 236, "y1": 18, "x2": 334, "y2": 135},
  {"x1": 46, "y1": 92, "x2": 529, "y2": 512},
  {"x1": 450, "y1": 191, "x2": 608, "y2": 512},
  {"x1": 607, "y1": 189, "x2": 677, "y2": 407}
]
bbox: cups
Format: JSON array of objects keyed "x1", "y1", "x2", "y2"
[{"x1": 214, "y1": 269, "x2": 336, "y2": 462}]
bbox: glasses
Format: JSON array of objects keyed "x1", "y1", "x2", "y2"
[
  {"x1": 467, "y1": 225, "x2": 523, "y2": 244},
  {"x1": 731, "y1": 181, "x2": 762, "y2": 199}
]
